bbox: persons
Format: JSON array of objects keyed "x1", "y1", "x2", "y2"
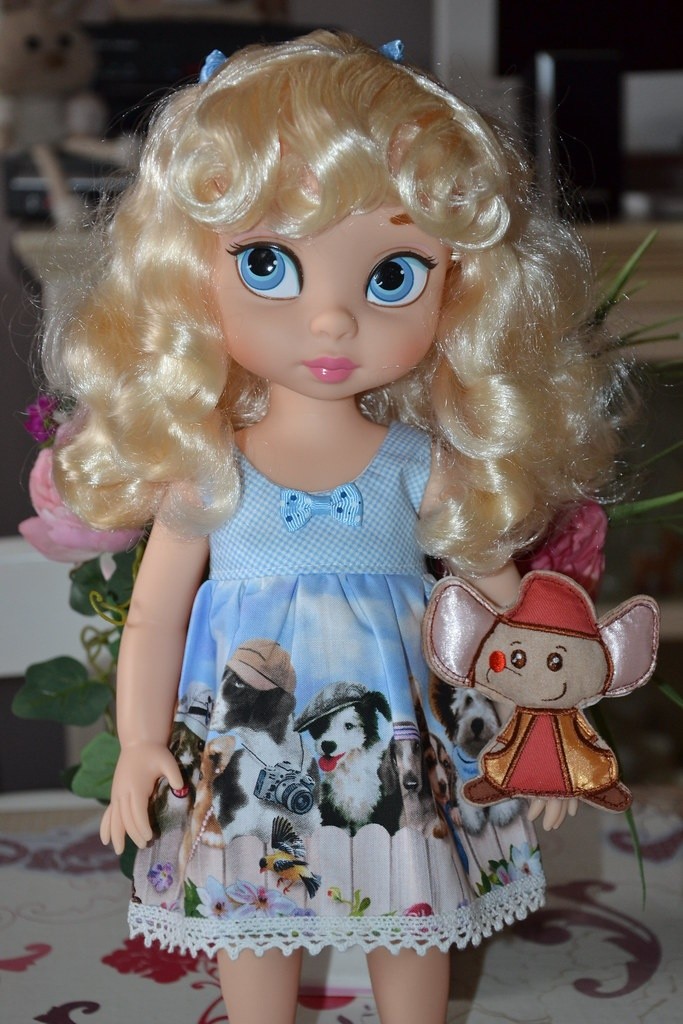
[
  {"x1": 0, "y1": 2, "x2": 90, "y2": 533},
  {"x1": 41, "y1": 32, "x2": 635, "y2": 1024}
]
